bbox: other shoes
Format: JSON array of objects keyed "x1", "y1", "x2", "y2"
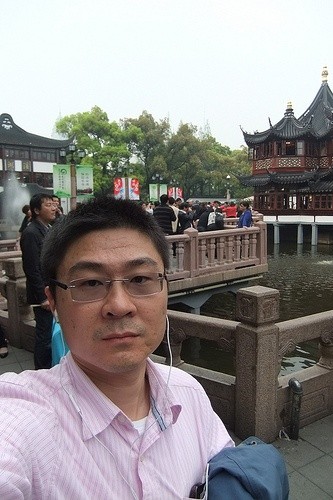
[{"x1": 0, "y1": 345, "x2": 8, "y2": 358}]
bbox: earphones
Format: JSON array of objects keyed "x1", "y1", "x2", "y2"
[{"x1": 54, "y1": 307, "x2": 60, "y2": 323}]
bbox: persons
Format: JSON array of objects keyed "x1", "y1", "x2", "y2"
[
  {"x1": 0, "y1": 325, "x2": 9, "y2": 358},
  {"x1": 0, "y1": 195, "x2": 237, "y2": 500},
  {"x1": 20, "y1": 194, "x2": 56, "y2": 369},
  {"x1": 19, "y1": 195, "x2": 63, "y2": 233},
  {"x1": 139, "y1": 194, "x2": 252, "y2": 260}
]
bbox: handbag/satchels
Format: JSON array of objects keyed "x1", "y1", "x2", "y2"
[{"x1": 51, "y1": 310, "x2": 69, "y2": 366}]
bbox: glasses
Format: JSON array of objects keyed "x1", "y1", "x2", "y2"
[{"x1": 51, "y1": 267, "x2": 168, "y2": 303}]
